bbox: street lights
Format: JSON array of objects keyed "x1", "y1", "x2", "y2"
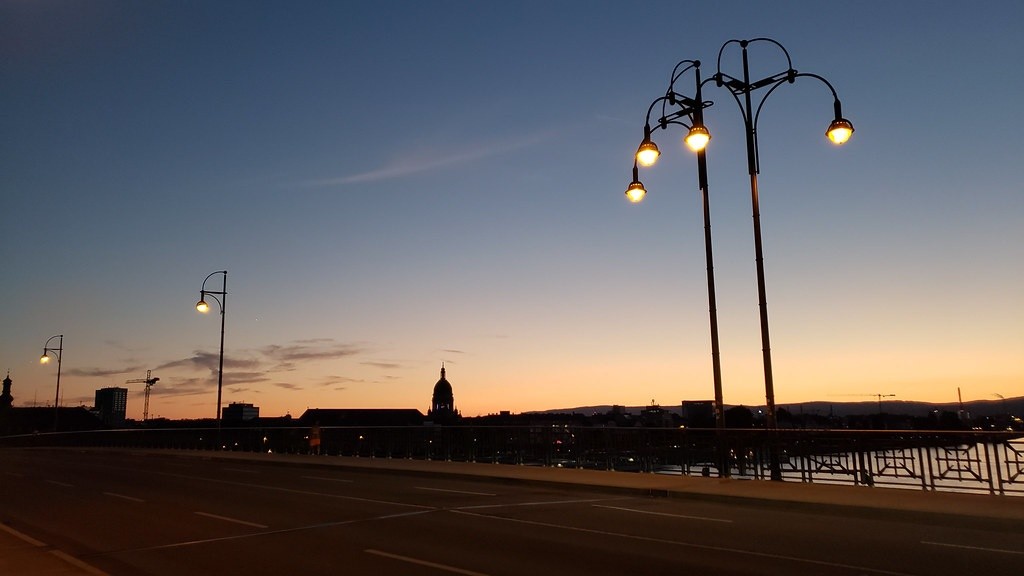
[
  {"x1": 685, "y1": 37, "x2": 854, "y2": 482},
  {"x1": 197, "y1": 271, "x2": 228, "y2": 450},
  {"x1": 40, "y1": 335, "x2": 64, "y2": 447},
  {"x1": 625, "y1": 60, "x2": 732, "y2": 479}
]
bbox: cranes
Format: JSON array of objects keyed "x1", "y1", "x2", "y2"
[{"x1": 126, "y1": 370, "x2": 160, "y2": 421}]
